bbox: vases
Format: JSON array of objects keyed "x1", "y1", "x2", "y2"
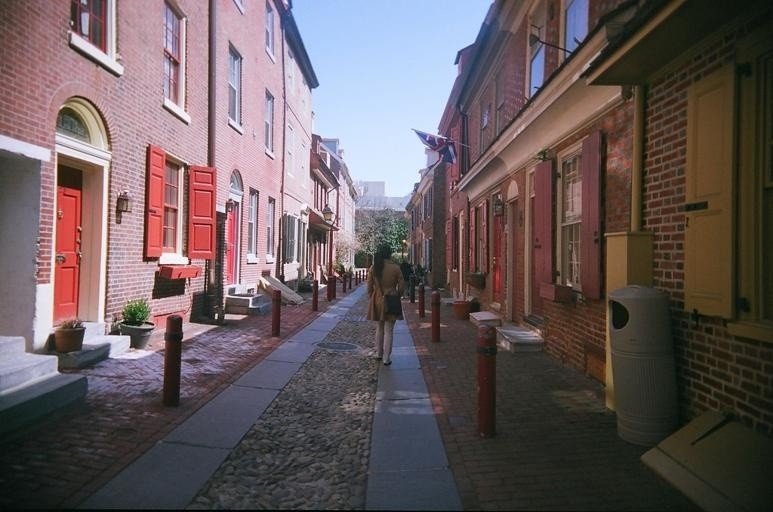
[{"x1": 454, "y1": 301, "x2": 470, "y2": 320}]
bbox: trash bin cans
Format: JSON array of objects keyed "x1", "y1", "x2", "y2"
[{"x1": 608, "y1": 284, "x2": 678, "y2": 446}]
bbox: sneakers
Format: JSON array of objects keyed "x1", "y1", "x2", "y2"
[{"x1": 374, "y1": 352, "x2": 392, "y2": 365}]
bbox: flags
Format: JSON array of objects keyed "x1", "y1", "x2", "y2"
[{"x1": 413, "y1": 129, "x2": 457, "y2": 166}]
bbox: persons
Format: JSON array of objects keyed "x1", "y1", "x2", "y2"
[
  {"x1": 364, "y1": 243, "x2": 405, "y2": 365},
  {"x1": 400, "y1": 258, "x2": 411, "y2": 300}
]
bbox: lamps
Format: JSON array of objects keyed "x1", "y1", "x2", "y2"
[
  {"x1": 322, "y1": 204, "x2": 334, "y2": 220},
  {"x1": 226, "y1": 198, "x2": 235, "y2": 213},
  {"x1": 493, "y1": 199, "x2": 504, "y2": 217},
  {"x1": 116, "y1": 187, "x2": 132, "y2": 212}
]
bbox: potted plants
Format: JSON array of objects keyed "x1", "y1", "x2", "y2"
[
  {"x1": 55, "y1": 318, "x2": 86, "y2": 352},
  {"x1": 119, "y1": 298, "x2": 155, "y2": 349}
]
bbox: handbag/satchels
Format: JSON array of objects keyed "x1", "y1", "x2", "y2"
[{"x1": 383, "y1": 294, "x2": 403, "y2": 315}]
